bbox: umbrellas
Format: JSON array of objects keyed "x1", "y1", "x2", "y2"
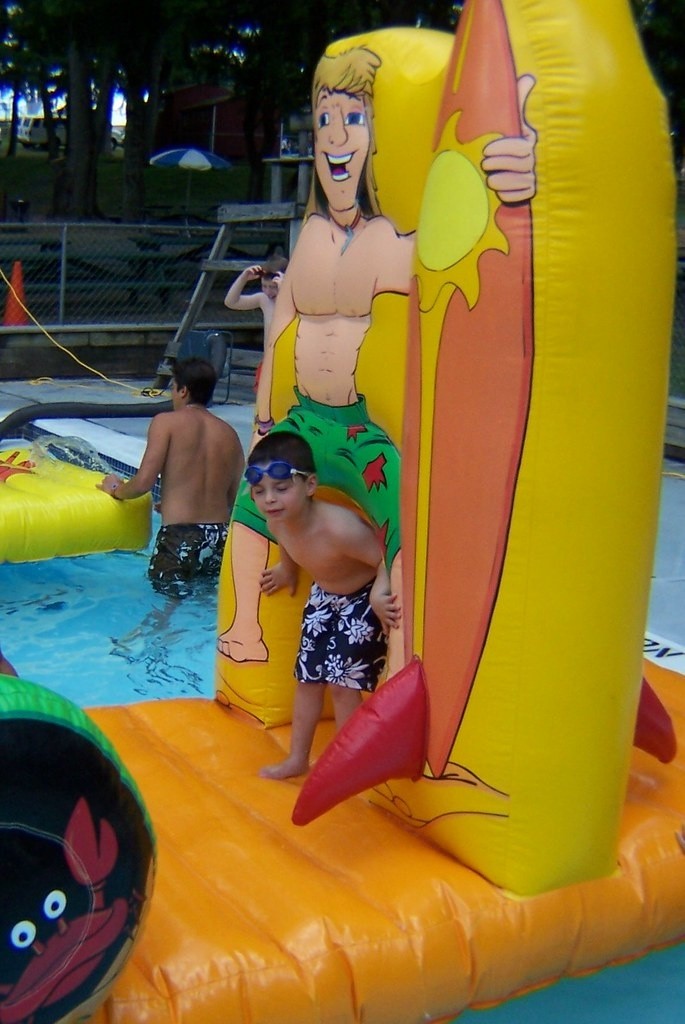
[{"x1": 148, "y1": 145, "x2": 232, "y2": 214}]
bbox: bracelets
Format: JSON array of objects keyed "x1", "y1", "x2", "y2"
[{"x1": 110, "y1": 481, "x2": 123, "y2": 498}]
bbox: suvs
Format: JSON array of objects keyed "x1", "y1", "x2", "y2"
[{"x1": 16, "y1": 115, "x2": 125, "y2": 151}]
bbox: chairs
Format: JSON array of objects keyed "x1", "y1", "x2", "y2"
[{"x1": 263, "y1": 113, "x2": 315, "y2": 206}]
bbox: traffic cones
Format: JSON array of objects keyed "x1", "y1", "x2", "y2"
[{"x1": 1, "y1": 261, "x2": 29, "y2": 326}]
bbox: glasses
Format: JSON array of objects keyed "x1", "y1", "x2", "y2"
[
  {"x1": 257, "y1": 270, "x2": 280, "y2": 279},
  {"x1": 243, "y1": 461, "x2": 312, "y2": 486}
]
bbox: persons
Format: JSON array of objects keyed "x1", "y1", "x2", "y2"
[
  {"x1": 246, "y1": 432, "x2": 401, "y2": 781},
  {"x1": 95, "y1": 358, "x2": 245, "y2": 599},
  {"x1": 224, "y1": 253, "x2": 289, "y2": 397}
]
